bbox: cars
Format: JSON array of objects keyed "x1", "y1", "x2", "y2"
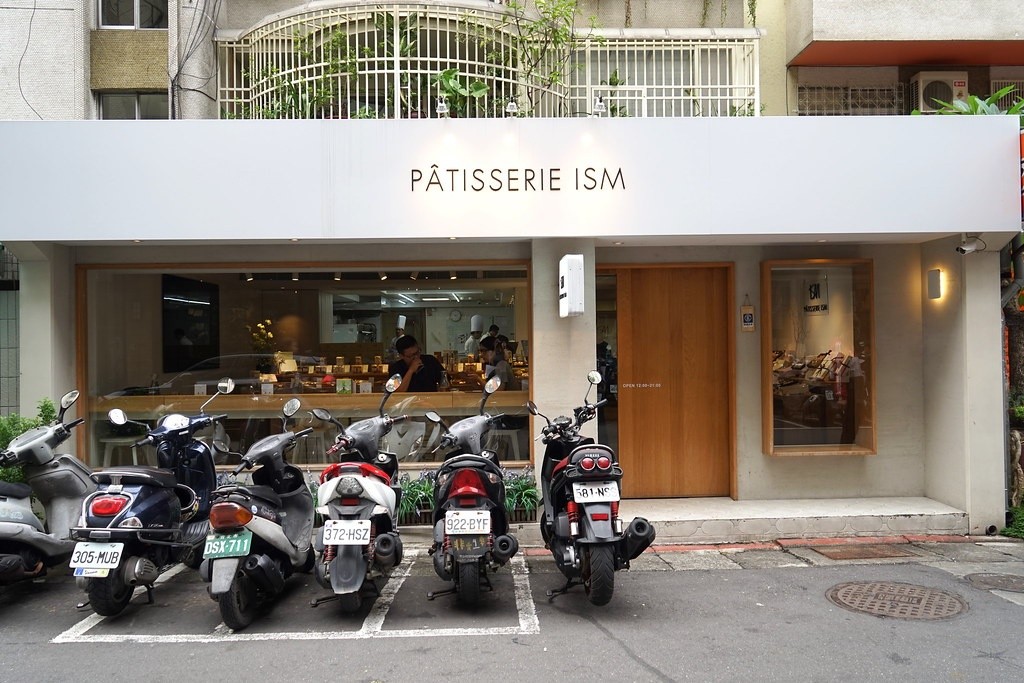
[{"x1": 104, "y1": 353, "x2": 327, "y2": 397}]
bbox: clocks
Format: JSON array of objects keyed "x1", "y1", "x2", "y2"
[{"x1": 449, "y1": 310, "x2": 462, "y2": 322}]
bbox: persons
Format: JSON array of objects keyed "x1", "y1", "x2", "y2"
[
  {"x1": 480, "y1": 324, "x2": 508, "y2": 369},
  {"x1": 389, "y1": 328, "x2": 405, "y2": 361},
  {"x1": 465, "y1": 331, "x2": 484, "y2": 354},
  {"x1": 389, "y1": 334, "x2": 445, "y2": 392},
  {"x1": 464, "y1": 336, "x2": 520, "y2": 391}
]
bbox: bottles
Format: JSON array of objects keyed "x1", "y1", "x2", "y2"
[
  {"x1": 291, "y1": 373, "x2": 303, "y2": 394},
  {"x1": 149, "y1": 374, "x2": 160, "y2": 395},
  {"x1": 439, "y1": 371, "x2": 451, "y2": 392}
]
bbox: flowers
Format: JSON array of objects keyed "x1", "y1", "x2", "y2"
[{"x1": 245, "y1": 319, "x2": 276, "y2": 371}]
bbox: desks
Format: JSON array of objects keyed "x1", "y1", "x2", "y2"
[{"x1": 89, "y1": 390, "x2": 528, "y2": 468}]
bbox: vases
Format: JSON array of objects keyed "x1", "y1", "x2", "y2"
[{"x1": 248, "y1": 368, "x2": 265, "y2": 393}]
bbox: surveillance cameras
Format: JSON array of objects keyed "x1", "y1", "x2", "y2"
[{"x1": 956, "y1": 242, "x2": 977, "y2": 255}]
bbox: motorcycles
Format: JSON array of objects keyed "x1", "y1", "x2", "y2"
[
  {"x1": 526, "y1": 369, "x2": 656, "y2": 607},
  {"x1": 426, "y1": 376, "x2": 519, "y2": 613},
  {"x1": 0, "y1": 388, "x2": 98, "y2": 600},
  {"x1": 381, "y1": 394, "x2": 441, "y2": 463},
  {"x1": 66, "y1": 374, "x2": 237, "y2": 616},
  {"x1": 310, "y1": 372, "x2": 430, "y2": 615},
  {"x1": 200, "y1": 397, "x2": 312, "y2": 630}
]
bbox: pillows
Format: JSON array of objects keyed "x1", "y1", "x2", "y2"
[{"x1": 299, "y1": 418, "x2": 324, "y2": 427}]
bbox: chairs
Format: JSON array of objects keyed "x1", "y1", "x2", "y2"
[
  {"x1": 485, "y1": 415, "x2": 521, "y2": 460},
  {"x1": 100, "y1": 419, "x2": 150, "y2": 467},
  {"x1": 289, "y1": 418, "x2": 334, "y2": 465}
]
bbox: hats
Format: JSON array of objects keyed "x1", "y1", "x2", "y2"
[
  {"x1": 470, "y1": 314, "x2": 483, "y2": 333},
  {"x1": 395, "y1": 315, "x2": 406, "y2": 331}
]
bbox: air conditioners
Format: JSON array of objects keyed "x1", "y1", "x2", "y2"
[{"x1": 910, "y1": 70, "x2": 970, "y2": 114}]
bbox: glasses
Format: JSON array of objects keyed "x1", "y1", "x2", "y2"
[
  {"x1": 402, "y1": 347, "x2": 422, "y2": 359},
  {"x1": 478, "y1": 349, "x2": 491, "y2": 354}
]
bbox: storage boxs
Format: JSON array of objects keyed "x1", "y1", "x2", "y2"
[{"x1": 811, "y1": 352, "x2": 854, "y2": 383}]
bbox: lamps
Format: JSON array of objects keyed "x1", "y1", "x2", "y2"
[
  {"x1": 245, "y1": 273, "x2": 253, "y2": 282},
  {"x1": 450, "y1": 271, "x2": 457, "y2": 279},
  {"x1": 334, "y1": 272, "x2": 341, "y2": 280},
  {"x1": 506, "y1": 96, "x2": 519, "y2": 118},
  {"x1": 928, "y1": 269, "x2": 943, "y2": 299},
  {"x1": 410, "y1": 271, "x2": 419, "y2": 279},
  {"x1": 593, "y1": 96, "x2": 606, "y2": 117},
  {"x1": 435, "y1": 95, "x2": 451, "y2": 119},
  {"x1": 292, "y1": 272, "x2": 298, "y2": 280},
  {"x1": 477, "y1": 271, "x2": 483, "y2": 279},
  {"x1": 378, "y1": 272, "x2": 387, "y2": 280}
]
